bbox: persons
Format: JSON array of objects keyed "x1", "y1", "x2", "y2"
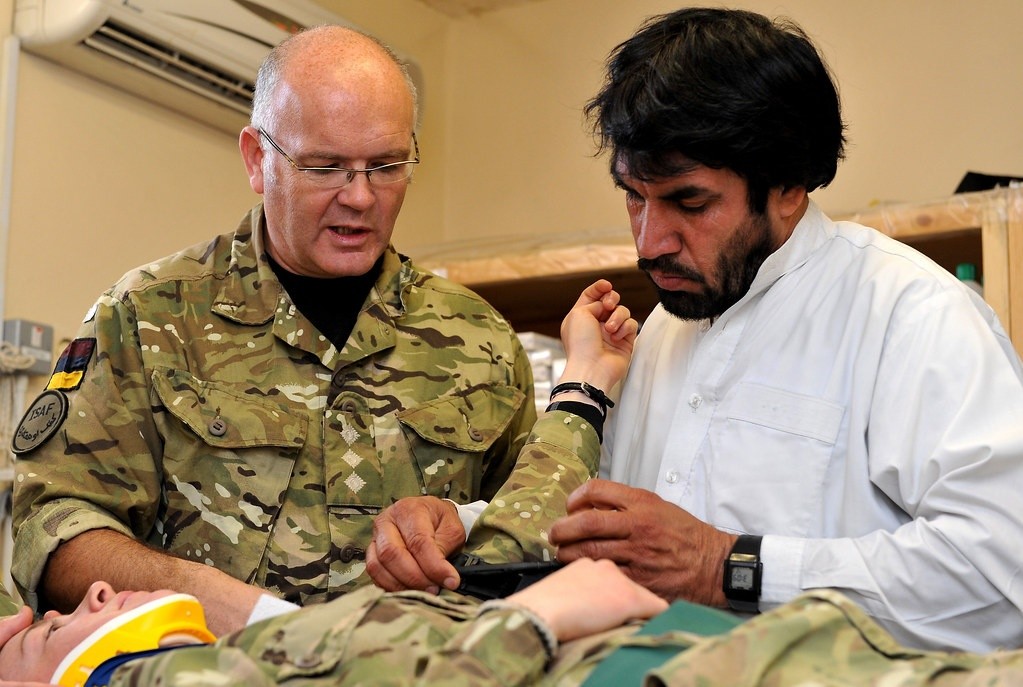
[
  {"x1": 0, "y1": 279, "x2": 1023, "y2": 687},
  {"x1": 10, "y1": 24, "x2": 536, "y2": 636},
  {"x1": 365, "y1": 5, "x2": 1023, "y2": 648}
]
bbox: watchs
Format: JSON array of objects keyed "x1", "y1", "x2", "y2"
[{"x1": 721, "y1": 535, "x2": 763, "y2": 615}]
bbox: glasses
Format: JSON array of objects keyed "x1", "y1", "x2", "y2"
[{"x1": 258, "y1": 127, "x2": 422, "y2": 189}]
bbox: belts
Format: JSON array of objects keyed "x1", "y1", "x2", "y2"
[{"x1": 582, "y1": 600, "x2": 743, "y2": 687}]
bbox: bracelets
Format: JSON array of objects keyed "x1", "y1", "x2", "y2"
[
  {"x1": 551, "y1": 379, "x2": 615, "y2": 422},
  {"x1": 475, "y1": 598, "x2": 559, "y2": 659}
]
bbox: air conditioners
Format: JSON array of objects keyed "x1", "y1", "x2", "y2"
[{"x1": 15, "y1": 0, "x2": 375, "y2": 138}]
